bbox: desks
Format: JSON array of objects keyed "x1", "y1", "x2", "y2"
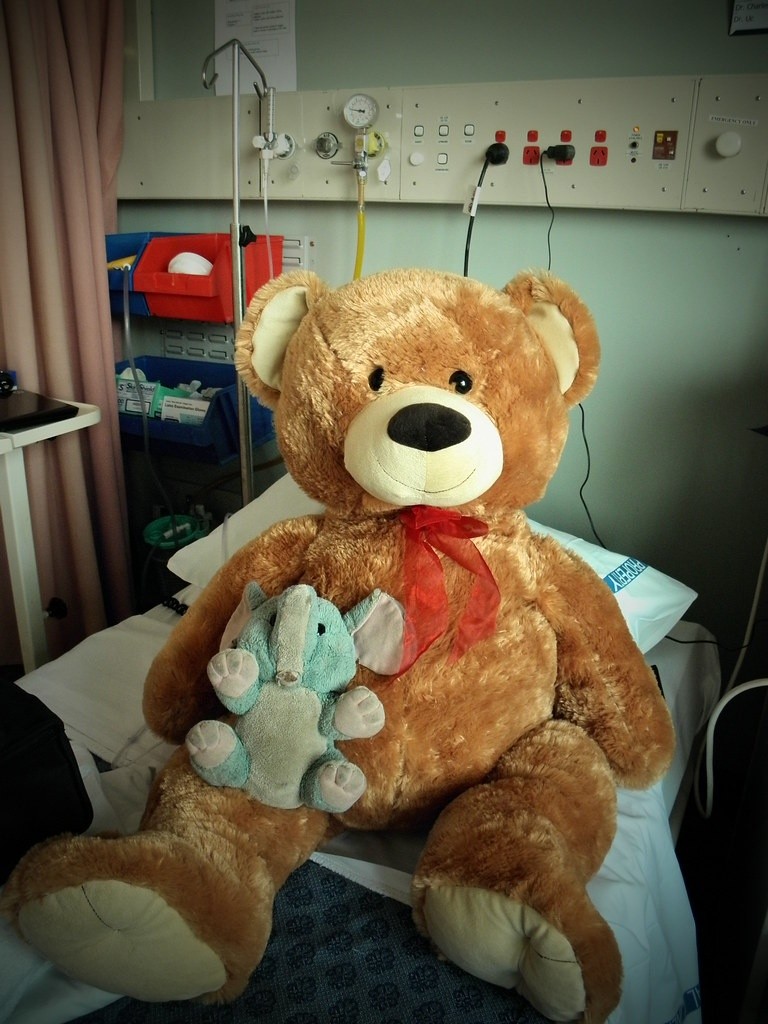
[{"x1": 0, "y1": 395, "x2": 103, "y2": 685}]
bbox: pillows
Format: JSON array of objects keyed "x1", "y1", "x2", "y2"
[{"x1": 166, "y1": 472, "x2": 699, "y2": 657}]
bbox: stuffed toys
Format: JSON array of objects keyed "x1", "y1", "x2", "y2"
[
  {"x1": 186, "y1": 579, "x2": 416, "y2": 814},
  {"x1": 1, "y1": 272, "x2": 675, "y2": 1022}
]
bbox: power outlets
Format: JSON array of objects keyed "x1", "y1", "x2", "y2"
[
  {"x1": 555, "y1": 146, "x2": 572, "y2": 165},
  {"x1": 523, "y1": 146, "x2": 540, "y2": 165},
  {"x1": 589, "y1": 146, "x2": 608, "y2": 166}
]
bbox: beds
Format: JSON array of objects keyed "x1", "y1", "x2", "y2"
[{"x1": 0, "y1": 471, "x2": 723, "y2": 1024}]
